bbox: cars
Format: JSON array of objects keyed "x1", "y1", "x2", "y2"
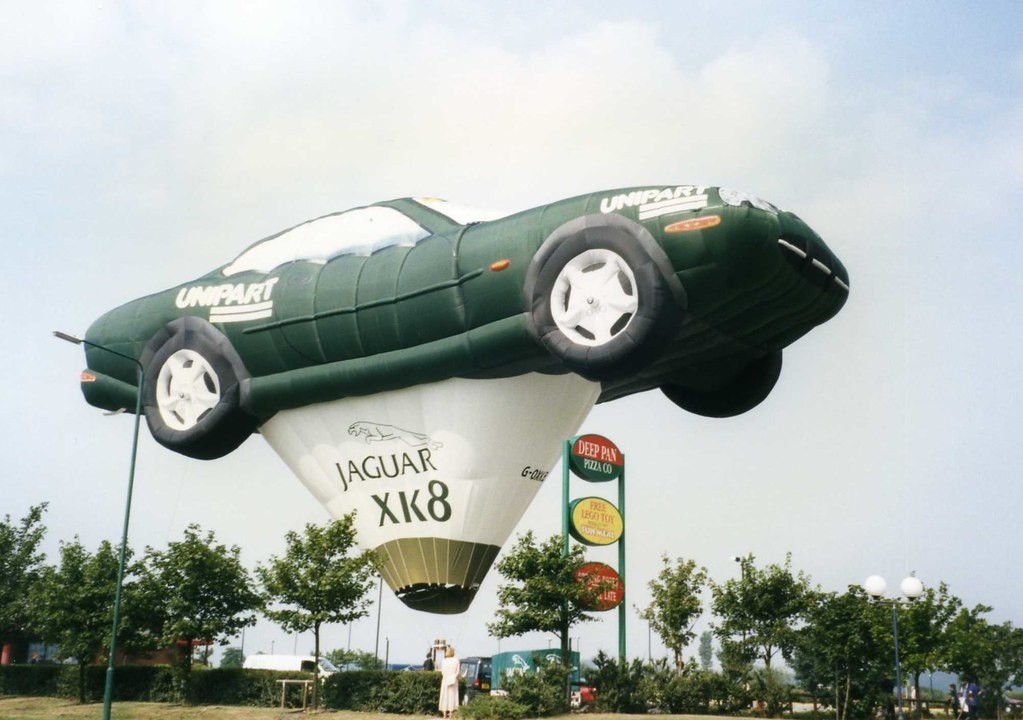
[
  {"x1": 580, "y1": 682, "x2": 599, "y2": 706},
  {"x1": 78, "y1": 184, "x2": 855, "y2": 461}
]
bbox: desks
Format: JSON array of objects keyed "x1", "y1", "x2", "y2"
[{"x1": 276, "y1": 679, "x2": 314, "y2": 709}]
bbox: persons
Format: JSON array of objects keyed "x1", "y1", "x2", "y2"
[
  {"x1": 28, "y1": 651, "x2": 38, "y2": 663},
  {"x1": 948, "y1": 681, "x2": 983, "y2": 720},
  {"x1": 424, "y1": 652, "x2": 435, "y2": 671},
  {"x1": 438, "y1": 648, "x2": 460, "y2": 718}
]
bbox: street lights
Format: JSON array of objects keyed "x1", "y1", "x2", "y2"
[
  {"x1": 375, "y1": 573, "x2": 384, "y2": 666},
  {"x1": 54, "y1": 329, "x2": 146, "y2": 719},
  {"x1": 865, "y1": 574, "x2": 923, "y2": 719},
  {"x1": 386, "y1": 636, "x2": 391, "y2": 671}
]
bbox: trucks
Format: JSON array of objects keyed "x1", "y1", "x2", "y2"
[
  {"x1": 242, "y1": 654, "x2": 340, "y2": 687},
  {"x1": 491, "y1": 647, "x2": 583, "y2": 710}
]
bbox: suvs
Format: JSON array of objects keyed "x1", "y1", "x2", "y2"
[{"x1": 458, "y1": 657, "x2": 494, "y2": 694}]
